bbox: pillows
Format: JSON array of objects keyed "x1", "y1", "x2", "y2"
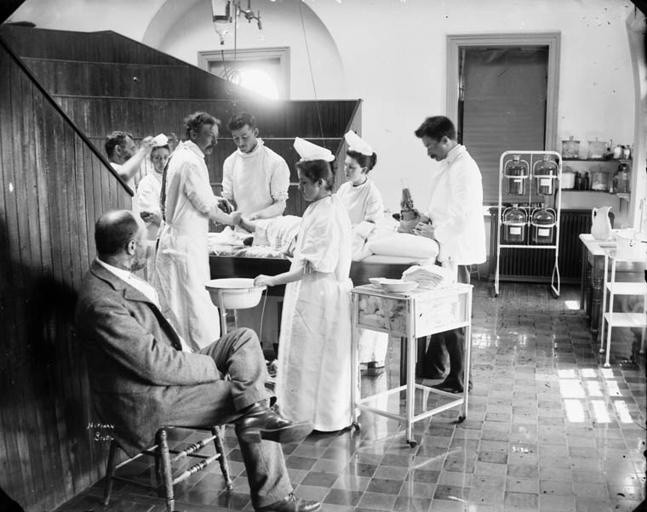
[{"x1": 366, "y1": 229, "x2": 440, "y2": 259}]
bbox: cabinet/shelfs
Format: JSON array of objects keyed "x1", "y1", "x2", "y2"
[
  {"x1": 599, "y1": 245, "x2": 647, "y2": 368},
  {"x1": 555, "y1": 157, "x2": 632, "y2": 215},
  {"x1": 494, "y1": 150, "x2": 563, "y2": 298}
]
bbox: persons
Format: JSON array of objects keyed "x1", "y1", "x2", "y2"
[
  {"x1": 336, "y1": 130, "x2": 389, "y2": 369},
  {"x1": 415, "y1": 116, "x2": 486, "y2": 394},
  {"x1": 234, "y1": 208, "x2": 432, "y2": 250},
  {"x1": 154, "y1": 111, "x2": 242, "y2": 352},
  {"x1": 237, "y1": 137, "x2": 361, "y2": 433},
  {"x1": 76, "y1": 208, "x2": 321, "y2": 512},
  {"x1": 222, "y1": 112, "x2": 290, "y2": 234},
  {"x1": 105, "y1": 131, "x2": 157, "y2": 182},
  {"x1": 132, "y1": 131, "x2": 178, "y2": 240}
]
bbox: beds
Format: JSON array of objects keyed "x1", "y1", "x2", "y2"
[{"x1": 206, "y1": 241, "x2": 437, "y2": 401}]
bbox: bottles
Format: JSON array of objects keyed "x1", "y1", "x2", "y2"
[
  {"x1": 583, "y1": 172, "x2": 589, "y2": 190},
  {"x1": 576, "y1": 173, "x2": 582, "y2": 189}
]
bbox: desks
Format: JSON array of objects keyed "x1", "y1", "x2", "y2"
[{"x1": 579, "y1": 228, "x2": 647, "y2": 340}]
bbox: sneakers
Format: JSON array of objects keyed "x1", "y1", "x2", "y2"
[
  {"x1": 416, "y1": 363, "x2": 444, "y2": 380},
  {"x1": 430, "y1": 382, "x2": 472, "y2": 394}
]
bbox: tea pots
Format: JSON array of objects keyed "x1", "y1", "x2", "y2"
[{"x1": 591, "y1": 206, "x2": 612, "y2": 241}]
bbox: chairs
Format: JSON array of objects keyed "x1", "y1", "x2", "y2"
[{"x1": 67, "y1": 325, "x2": 233, "y2": 512}]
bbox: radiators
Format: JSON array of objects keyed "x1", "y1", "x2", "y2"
[{"x1": 487, "y1": 206, "x2": 616, "y2": 286}]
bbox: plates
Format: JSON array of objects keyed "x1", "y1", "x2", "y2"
[{"x1": 381, "y1": 280, "x2": 417, "y2": 292}]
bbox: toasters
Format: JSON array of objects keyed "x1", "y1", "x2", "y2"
[{"x1": 621, "y1": 166, "x2": 628, "y2": 193}]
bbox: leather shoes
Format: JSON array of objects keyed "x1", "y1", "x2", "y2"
[
  {"x1": 236, "y1": 409, "x2": 316, "y2": 444},
  {"x1": 256, "y1": 492, "x2": 322, "y2": 512}
]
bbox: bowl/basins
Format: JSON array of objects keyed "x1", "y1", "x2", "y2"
[{"x1": 206, "y1": 278, "x2": 266, "y2": 310}]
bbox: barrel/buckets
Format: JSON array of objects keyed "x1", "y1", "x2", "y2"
[
  {"x1": 503, "y1": 204, "x2": 526, "y2": 243},
  {"x1": 532, "y1": 202, "x2": 554, "y2": 243},
  {"x1": 535, "y1": 154, "x2": 556, "y2": 197},
  {"x1": 505, "y1": 155, "x2": 526, "y2": 195}
]
